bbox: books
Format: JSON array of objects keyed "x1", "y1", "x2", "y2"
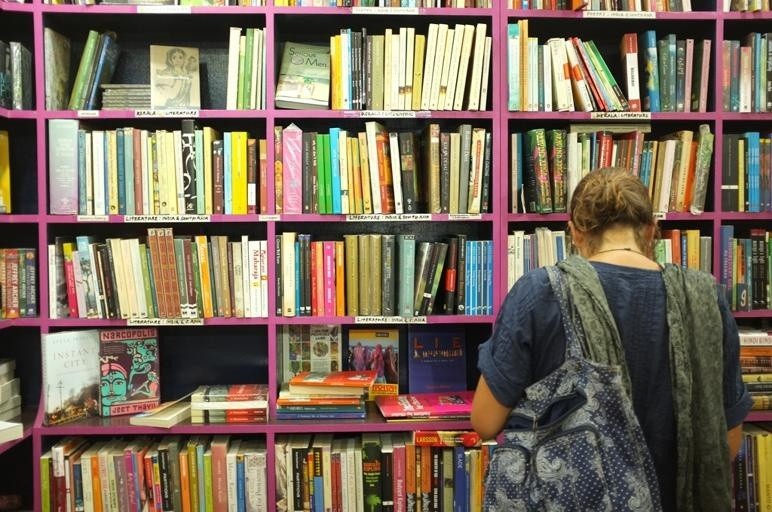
[
  {"x1": 507, "y1": 0, "x2": 772, "y2": 114},
  {"x1": 727, "y1": 324, "x2": 772, "y2": 511},
  {"x1": 0, "y1": 358, "x2": 25, "y2": 443},
  {"x1": 48, "y1": 119, "x2": 270, "y2": 319},
  {"x1": 276, "y1": 122, "x2": 494, "y2": 315},
  {"x1": 275, "y1": 324, "x2": 498, "y2": 512},
  {"x1": 0, "y1": 0, "x2": 34, "y2": 110},
  {"x1": 508, "y1": 125, "x2": 769, "y2": 310},
  {"x1": 43, "y1": 1, "x2": 266, "y2": 111},
  {"x1": 275, "y1": 1, "x2": 493, "y2": 109},
  {"x1": 41, "y1": 329, "x2": 268, "y2": 512},
  {"x1": 0, "y1": 131, "x2": 21, "y2": 214},
  {"x1": 0, "y1": 248, "x2": 37, "y2": 317}
]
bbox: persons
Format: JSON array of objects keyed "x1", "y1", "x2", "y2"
[{"x1": 468, "y1": 170, "x2": 754, "y2": 511}]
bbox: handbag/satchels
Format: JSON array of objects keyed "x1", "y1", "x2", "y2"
[{"x1": 483, "y1": 359, "x2": 663, "y2": 512}]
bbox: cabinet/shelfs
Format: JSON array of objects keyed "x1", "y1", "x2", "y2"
[{"x1": 0, "y1": 0, "x2": 772, "y2": 512}]
